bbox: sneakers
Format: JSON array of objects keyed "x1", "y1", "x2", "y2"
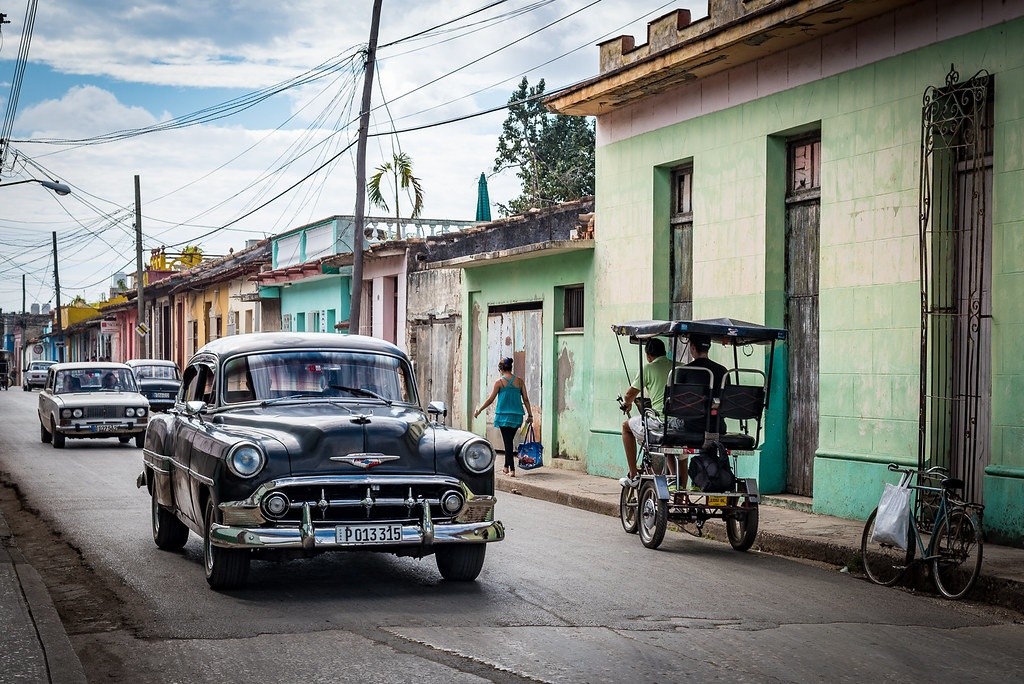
[
  {"x1": 619, "y1": 473, "x2": 640, "y2": 488},
  {"x1": 666, "y1": 477, "x2": 677, "y2": 486}
]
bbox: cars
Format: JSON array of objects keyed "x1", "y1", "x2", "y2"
[
  {"x1": 22, "y1": 361, "x2": 59, "y2": 392},
  {"x1": 124, "y1": 359, "x2": 183, "y2": 413},
  {"x1": 36, "y1": 362, "x2": 151, "y2": 449},
  {"x1": 134, "y1": 331, "x2": 507, "y2": 592}
]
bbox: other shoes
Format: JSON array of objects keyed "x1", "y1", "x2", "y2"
[
  {"x1": 503, "y1": 469, "x2": 509, "y2": 475},
  {"x1": 507, "y1": 472, "x2": 515, "y2": 477}
]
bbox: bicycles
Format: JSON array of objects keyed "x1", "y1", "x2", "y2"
[
  {"x1": 8, "y1": 376, "x2": 14, "y2": 388},
  {"x1": 859, "y1": 461, "x2": 988, "y2": 601}
]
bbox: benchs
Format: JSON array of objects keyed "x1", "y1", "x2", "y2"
[{"x1": 648, "y1": 366, "x2": 767, "y2": 450}]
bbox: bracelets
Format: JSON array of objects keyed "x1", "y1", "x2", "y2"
[{"x1": 528, "y1": 415, "x2": 533, "y2": 418}]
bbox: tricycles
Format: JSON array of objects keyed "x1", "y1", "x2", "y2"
[
  {"x1": 609, "y1": 317, "x2": 789, "y2": 551},
  {"x1": 0, "y1": 349, "x2": 10, "y2": 391}
]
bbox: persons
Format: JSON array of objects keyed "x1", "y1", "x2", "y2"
[
  {"x1": 101, "y1": 373, "x2": 124, "y2": 390},
  {"x1": 87, "y1": 356, "x2": 110, "y2": 362},
  {"x1": 245, "y1": 368, "x2": 272, "y2": 399},
  {"x1": 617, "y1": 338, "x2": 686, "y2": 490},
  {"x1": 474, "y1": 357, "x2": 534, "y2": 476},
  {"x1": 667, "y1": 334, "x2": 731, "y2": 490},
  {"x1": 63, "y1": 375, "x2": 73, "y2": 392}
]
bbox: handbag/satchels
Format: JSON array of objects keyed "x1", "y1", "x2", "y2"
[
  {"x1": 688, "y1": 442, "x2": 736, "y2": 493},
  {"x1": 518, "y1": 422, "x2": 544, "y2": 469},
  {"x1": 870, "y1": 472, "x2": 913, "y2": 550}
]
